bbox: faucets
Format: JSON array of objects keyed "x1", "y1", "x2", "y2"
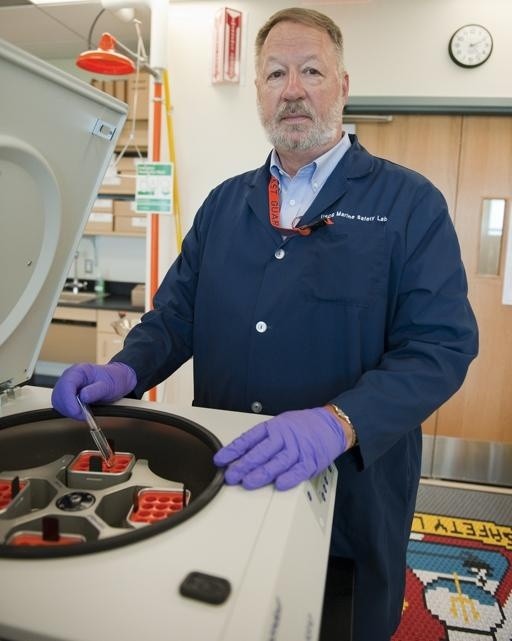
[{"x1": 63, "y1": 250, "x2": 88, "y2": 293}]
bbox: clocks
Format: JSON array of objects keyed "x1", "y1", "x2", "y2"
[{"x1": 448, "y1": 24, "x2": 493, "y2": 67}]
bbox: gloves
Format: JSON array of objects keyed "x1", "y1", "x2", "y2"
[
  {"x1": 52, "y1": 362, "x2": 137, "y2": 419},
  {"x1": 212, "y1": 405, "x2": 348, "y2": 491}
]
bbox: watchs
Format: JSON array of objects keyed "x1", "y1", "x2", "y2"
[{"x1": 332, "y1": 404, "x2": 357, "y2": 446}]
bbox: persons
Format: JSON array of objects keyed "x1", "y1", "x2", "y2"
[{"x1": 51, "y1": 8, "x2": 478, "y2": 641}]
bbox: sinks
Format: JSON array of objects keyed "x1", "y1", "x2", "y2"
[{"x1": 57, "y1": 292, "x2": 109, "y2": 305}]
